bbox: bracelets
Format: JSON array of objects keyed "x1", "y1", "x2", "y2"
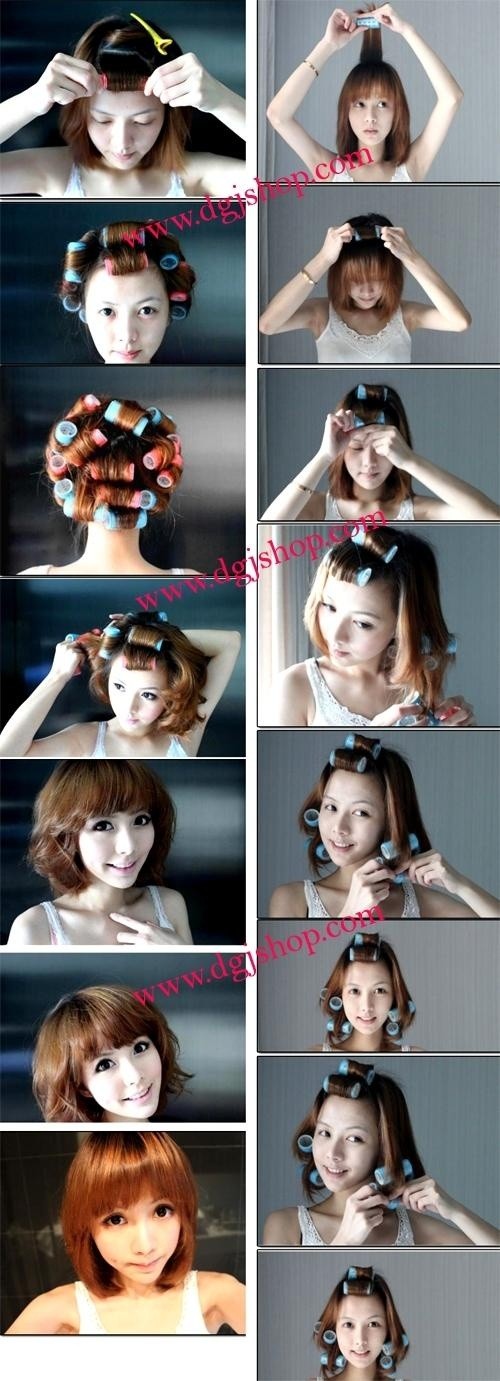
[
  {"x1": 303, "y1": 57, "x2": 320, "y2": 78},
  {"x1": 298, "y1": 265, "x2": 317, "y2": 289},
  {"x1": 289, "y1": 478, "x2": 316, "y2": 499}
]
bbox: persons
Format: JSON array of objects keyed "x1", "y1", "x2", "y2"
[
  {"x1": 312, "y1": 1264, "x2": 409, "y2": 1381},
  {"x1": 263, "y1": 1058, "x2": 499, "y2": 1248},
  {"x1": 1, "y1": 1133, "x2": 247, "y2": 1336},
  {"x1": 58, "y1": 217, "x2": 197, "y2": 366},
  {"x1": 259, "y1": 214, "x2": 470, "y2": 365},
  {"x1": 266, "y1": 2, "x2": 464, "y2": 183},
  {"x1": 261, "y1": 527, "x2": 479, "y2": 725},
  {"x1": 1, "y1": 609, "x2": 241, "y2": 758},
  {"x1": 267, "y1": 735, "x2": 499, "y2": 918},
  {"x1": 307, "y1": 930, "x2": 428, "y2": 1055},
  {"x1": 259, "y1": 381, "x2": 498, "y2": 520},
  {"x1": 28, "y1": 983, "x2": 198, "y2": 1123},
  {"x1": 18, "y1": 389, "x2": 203, "y2": 575},
  {"x1": 7, "y1": 760, "x2": 197, "y2": 945},
  {"x1": 0, "y1": 13, "x2": 247, "y2": 198}
]
